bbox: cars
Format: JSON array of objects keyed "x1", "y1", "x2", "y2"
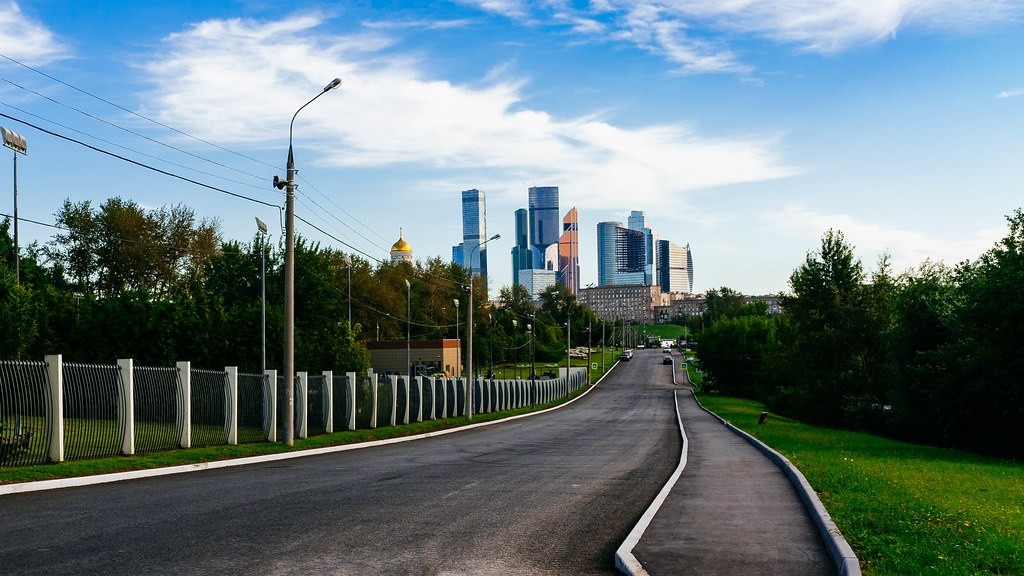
[
  {"x1": 662, "y1": 345, "x2": 672, "y2": 353},
  {"x1": 662, "y1": 356, "x2": 673, "y2": 365},
  {"x1": 637, "y1": 342, "x2": 647, "y2": 350}
]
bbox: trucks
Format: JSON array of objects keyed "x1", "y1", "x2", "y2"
[{"x1": 621, "y1": 350, "x2": 634, "y2": 361}]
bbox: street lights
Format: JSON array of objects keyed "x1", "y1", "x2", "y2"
[
  {"x1": 1, "y1": 125, "x2": 27, "y2": 438},
  {"x1": 404, "y1": 278, "x2": 412, "y2": 377},
  {"x1": 284, "y1": 77, "x2": 343, "y2": 450},
  {"x1": 512, "y1": 319, "x2": 517, "y2": 380},
  {"x1": 454, "y1": 299, "x2": 460, "y2": 380},
  {"x1": 566, "y1": 283, "x2": 595, "y2": 400},
  {"x1": 527, "y1": 324, "x2": 531, "y2": 380},
  {"x1": 465, "y1": 234, "x2": 501, "y2": 422},
  {"x1": 255, "y1": 217, "x2": 267, "y2": 435},
  {"x1": 488, "y1": 313, "x2": 492, "y2": 382}
]
